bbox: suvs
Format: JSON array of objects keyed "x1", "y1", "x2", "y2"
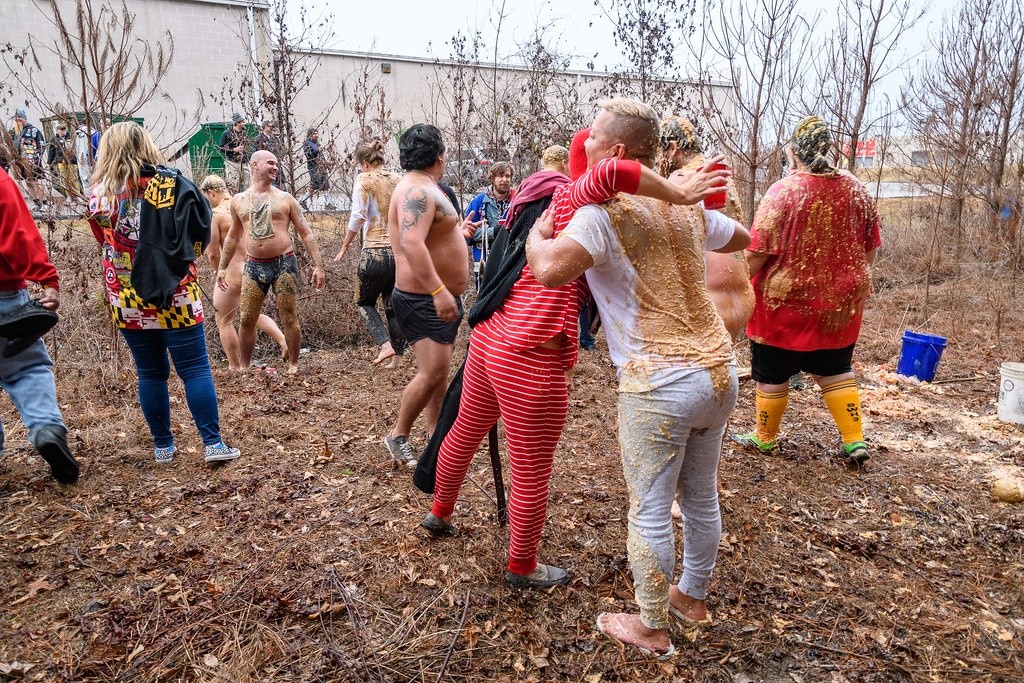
[{"x1": 441, "y1": 147, "x2": 513, "y2": 190}]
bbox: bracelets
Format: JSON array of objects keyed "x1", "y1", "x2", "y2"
[{"x1": 431, "y1": 283, "x2": 446, "y2": 297}]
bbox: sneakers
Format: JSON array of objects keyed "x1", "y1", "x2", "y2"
[
  {"x1": 29, "y1": 205, "x2": 41, "y2": 212},
  {"x1": 35, "y1": 425, "x2": 80, "y2": 484},
  {"x1": 384, "y1": 429, "x2": 418, "y2": 467},
  {"x1": 729, "y1": 429, "x2": 780, "y2": 454},
  {"x1": 420, "y1": 514, "x2": 458, "y2": 538},
  {"x1": 426, "y1": 432, "x2": 431, "y2": 446},
  {"x1": 507, "y1": 563, "x2": 570, "y2": 586},
  {"x1": 154, "y1": 441, "x2": 177, "y2": 463},
  {"x1": 837, "y1": 441, "x2": 870, "y2": 463},
  {"x1": 40, "y1": 205, "x2": 53, "y2": 212},
  {"x1": 204, "y1": 441, "x2": 241, "y2": 462}
]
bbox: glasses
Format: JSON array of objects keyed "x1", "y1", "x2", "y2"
[{"x1": 61, "y1": 129, "x2": 65, "y2": 130}]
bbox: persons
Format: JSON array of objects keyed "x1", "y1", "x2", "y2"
[
  {"x1": 196, "y1": 175, "x2": 288, "y2": 371},
  {"x1": 215, "y1": 150, "x2": 325, "y2": 381},
  {"x1": 421, "y1": 97, "x2": 757, "y2": 660},
  {"x1": 332, "y1": 140, "x2": 407, "y2": 370},
  {"x1": 0, "y1": 164, "x2": 84, "y2": 484},
  {"x1": 218, "y1": 112, "x2": 384, "y2": 212},
  {"x1": 383, "y1": 121, "x2": 489, "y2": 472},
  {"x1": 85, "y1": 122, "x2": 243, "y2": 464},
  {"x1": 7, "y1": 109, "x2": 115, "y2": 213},
  {"x1": 727, "y1": 116, "x2": 882, "y2": 465}
]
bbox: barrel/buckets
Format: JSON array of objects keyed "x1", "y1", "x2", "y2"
[
  {"x1": 998, "y1": 362, "x2": 1024, "y2": 424},
  {"x1": 896, "y1": 330, "x2": 947, "y2": 382}
]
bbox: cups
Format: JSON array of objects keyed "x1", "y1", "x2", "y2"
[
  {"x1": 498, "y1": 219, "x2": 506, "y2": 224},
  {"x1": 696, "y1": 163, "x2": 728, "y2": 210}
]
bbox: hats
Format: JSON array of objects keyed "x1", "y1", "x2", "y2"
[
  {"x1": 233, "y1": 113, "x2": 245, "y2": 123},
  {"x1": 57, "y1": 125, "x2": 66, "y2": 130},
  {"x1": 14, "y1": 111, "x2": 27, "y2": 120}
]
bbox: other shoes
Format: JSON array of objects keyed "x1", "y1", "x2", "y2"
[
  {"x1": 580, "y1": 334, "x2": 595, "y2": 351},
  {"x1": 299, "y1": 199, "x2": 310, "y2": 211},
  {"x1": 669, "y1": 604, "x2": 711, "y2": 627},
  {"x1": 0, "y1": 298, "x2": 58, "y2": 358},
  {"x1": 597, "y1": 612, "x2": 674, "y2": 660},
  {"x1": 324, "y1": 203, "x2": 338, "y2": 210}
]
